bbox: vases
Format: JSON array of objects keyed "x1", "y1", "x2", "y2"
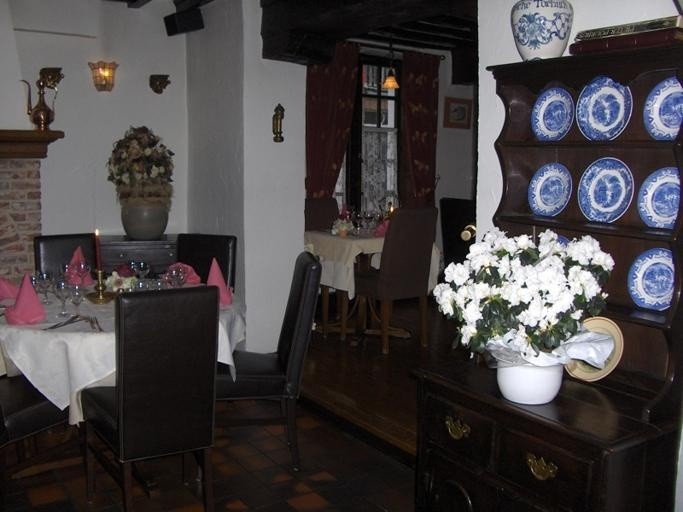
[
  {"x1": 511, "y1": 0, "x2": 573, "y2": 62},
  {"x1": 496, "y1": 361, "x2": 564, "y2": 405},
  {"x1": 116, "y1": 187, "x2": 173, "y2": 240}
]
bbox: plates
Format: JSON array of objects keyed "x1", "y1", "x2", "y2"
[
  {"x1": 564, "y1": 316, "x2": 624, "y2": 382},
  {"x1": 527, "y1": 74, "x2": 678, "y2": 313}
]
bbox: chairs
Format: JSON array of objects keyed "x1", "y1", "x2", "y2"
[
  {"x1": 305, "y1": 196, "x2": 346, "y2": 322},
  {"x1": 440, "y1": 197, "x2": 476, "y2": 271},
  {"x1": 355, "y1": 205, "x2": 438, "y2": 353}
]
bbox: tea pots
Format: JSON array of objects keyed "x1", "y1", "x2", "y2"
[{"x1": 18, "y1": 78, "x2": 59, "y2": 131}]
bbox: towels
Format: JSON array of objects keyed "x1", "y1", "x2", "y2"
[{"x1": 374, "y1": 220, "x2": 390, "y2": 238}]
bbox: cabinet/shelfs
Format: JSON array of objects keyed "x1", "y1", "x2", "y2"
[{"x1": 409, "y1": 42, "x2": 683, "y2": 512}]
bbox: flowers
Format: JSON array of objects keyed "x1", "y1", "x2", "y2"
[
  {"x1": 105, "y1": 126, "x2": 174, "y2": 188},
  {"x1": 433, "y1": 230, "x2": 619, "y2": 364}
]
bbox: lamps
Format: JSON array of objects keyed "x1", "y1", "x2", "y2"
[
  {"x1": 381, "y1": 35, "x2": 400, "y2": 89},
  {"x1": 88, "y1": 60, "x2": 119, "y2": 92}
]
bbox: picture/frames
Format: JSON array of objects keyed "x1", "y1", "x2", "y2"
[{"x1": 443, "y1": 96, "x2": 472, "y2": 129}]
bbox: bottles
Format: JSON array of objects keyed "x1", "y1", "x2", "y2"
[{"x1": 117, "y1": 288, "x2": 133, "y2": 296}]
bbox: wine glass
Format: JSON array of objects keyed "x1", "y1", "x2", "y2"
[
  {"x1": 129, "y1": 259, "x2": 188, "y2": 291},
  {"x1": 31, "y1": 260, "x2": 91, "y2": 318},
  {"x1": 351, "y1": 209, "x2": 380, "y2": 237}
]
